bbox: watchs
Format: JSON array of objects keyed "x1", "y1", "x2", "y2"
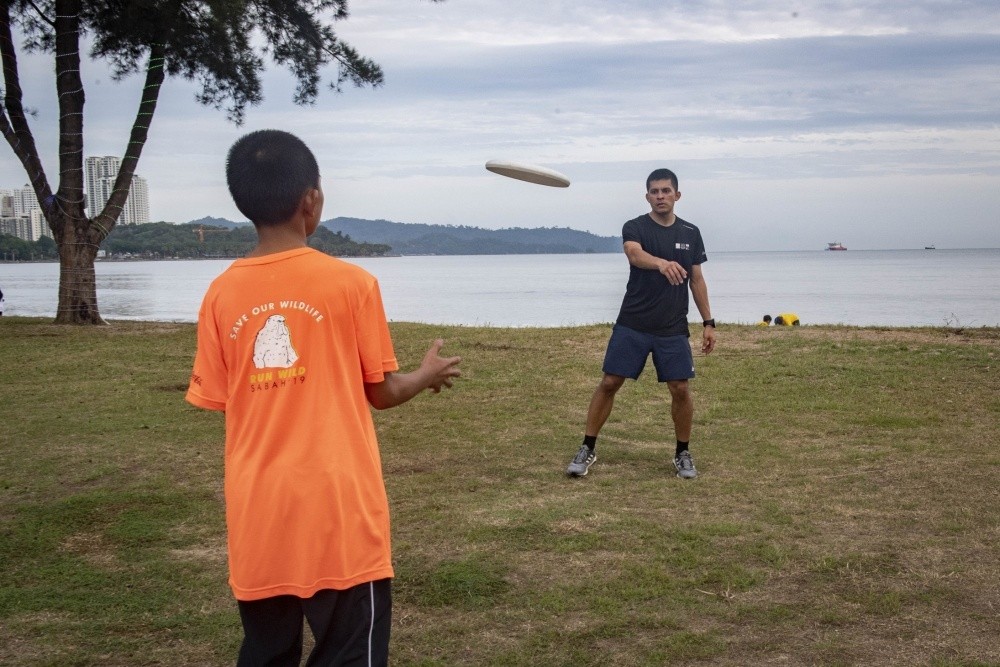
[{"x1": 702, "y1": 319, "x2": 716, "y2": 328}]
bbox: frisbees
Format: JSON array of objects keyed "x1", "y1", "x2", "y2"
[{"x1": 484, "y1": 159, "x2": 571, "y2": 189}]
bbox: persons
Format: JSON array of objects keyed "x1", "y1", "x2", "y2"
[
  {"x1": 566, "y1": 168, "x2": 716, "y2": 479},
  {"x1": 184, "y1": 129, "x2": 460, "y2": 667},
  {"x1": 774, "y1": 313, "x2": 801, "y2": 326},
  {"x1": 757, "y1": 315, "x2": 772, "y2": 327}
]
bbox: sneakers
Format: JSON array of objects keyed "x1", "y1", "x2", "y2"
[
  {"x1": 566, "y1": 445, "x2": 596, "y2": 477},
  {"x1": 672, "y1": 450, "x2": 698, "y2": 479}
]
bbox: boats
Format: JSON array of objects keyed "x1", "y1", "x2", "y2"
[
  {"x1": 926, "y1": 244, "x2": 936, "y2": 249},
  {"x1": 827, "y1": 243, "x2": 848, "y2": 251}
]
